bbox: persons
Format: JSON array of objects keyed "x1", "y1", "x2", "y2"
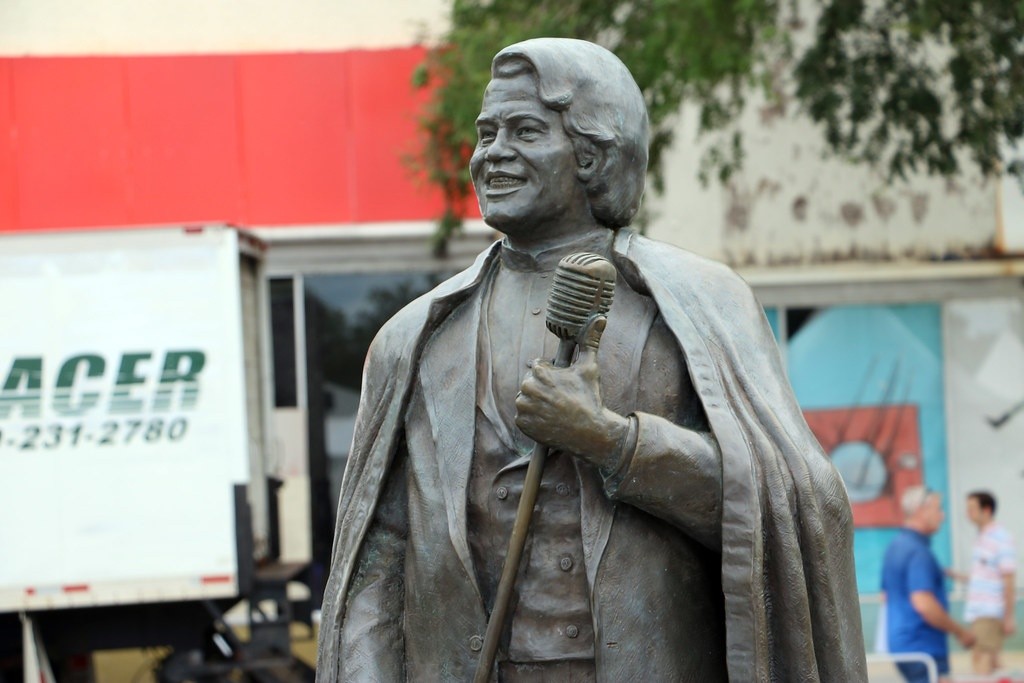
[
  {"x1": 313, "y1": 37, "x2": 869, "y2": 683},
  {"x1": 880, "y1": 485, "x2": 974, "y2": 683},
  {"x1": 943, "y1": 489, "x2": 1019, "y2": 672}
]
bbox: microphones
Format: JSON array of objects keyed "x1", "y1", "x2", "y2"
[{"x1": 545, "y1": 251, "x2": 616, "y2": 368}]
